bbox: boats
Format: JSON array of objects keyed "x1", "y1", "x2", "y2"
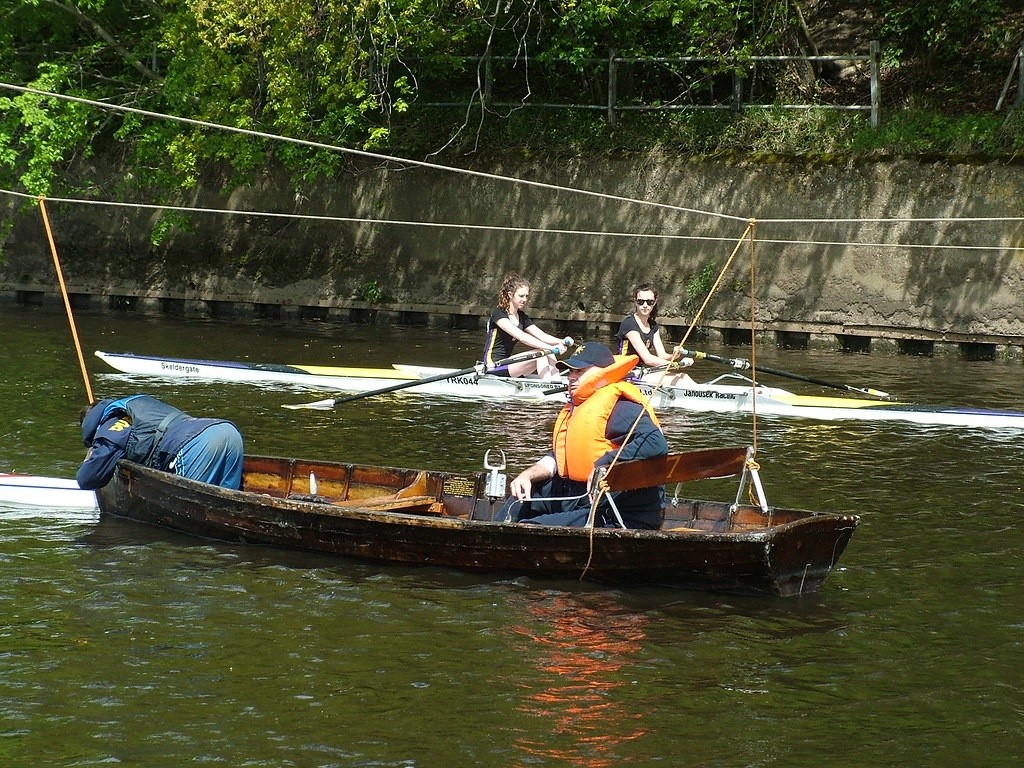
[
  {"x1": 95, "y1": 357, "x2": 1024, "y2": 434},
  {"x1": 95, "y1": 453, "x2": 862, "y2": 601},
  {"x1": 0, "y1": 472, "x2": 100, "y2": 510}
]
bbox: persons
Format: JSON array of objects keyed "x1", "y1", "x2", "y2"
[
  {"x1": 484, "y1": 271, "x2": 574, "y2": 383},
  {"x1": 76, "y1": 395, "x2": 243, "y2": 492},
  {"x1": 495, "y1": 342, "x2": 669, "y2": 532},
  {"x1": 618, "y1": 282, "x2": 698, "y2": 386}
]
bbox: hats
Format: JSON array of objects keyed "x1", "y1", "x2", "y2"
[{"x1": 555, "y1": 342, "x2": 616, "y2": 370}]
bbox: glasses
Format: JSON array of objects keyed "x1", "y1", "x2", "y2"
[{"x1": 636, "y1": 299, "x2": 655, "y2": 305}]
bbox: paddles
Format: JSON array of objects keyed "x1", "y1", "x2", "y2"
[
  {"x1": 565, "y1": 339, "x2": 579, "y2": 348},
  {"x1": 280, "y1": 347, "x2": 559, "y2": 410},
  {"x1": 673, "y1": 344, "x2": 890, "y2": 397},
  {"x1": 499, "y1": 361, "x2": 685, "y2": 402}
]
